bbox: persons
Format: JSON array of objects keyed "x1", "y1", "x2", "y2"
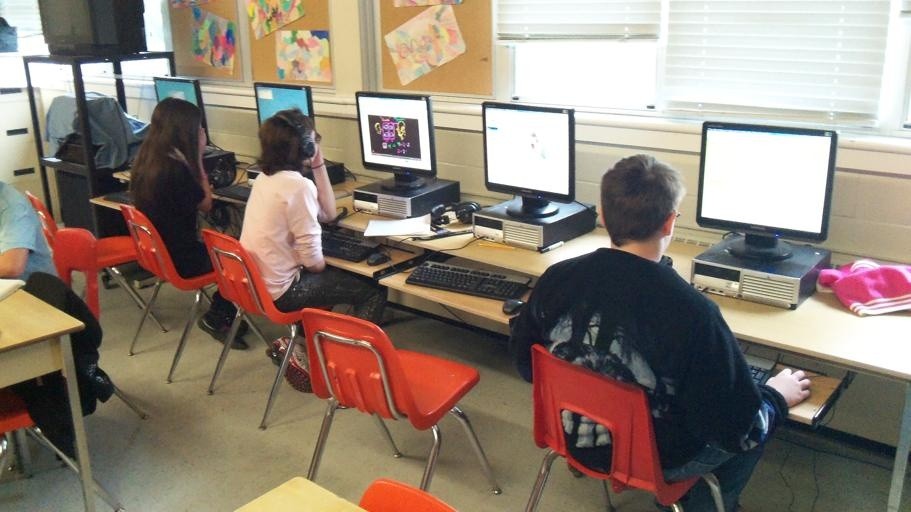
[
  {"x1": 507, "y1": 155, "x2": 810, "y2": 511},
  {"x1": 1, "y1": 180, "x2": 59, "y2": 277},
  {"x1": 133, "y1": 96, "x2": 249, "y2": 352},
  {"x1": 239, "y1": 109, "x2": 385, "y2": 395}
]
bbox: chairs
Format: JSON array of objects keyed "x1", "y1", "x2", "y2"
[
  {"x1": 525, "y1": 339, "x2": 735, "y2": 512},
  {"x1": 358, "y1": 479, "x2": 459, "y2": 512},
  {"x1": 24, "y1": 187, "x2": 167, "y2": 334},
  {"x1": 200, "y1": 225, "x2": 334, "y2": 431},
  {"x1": 299, "y1": 302, "x2": 506, "y2": 501},
  {"x1": 118, "y1": 201, "x2": 271, "y2": 384},
  {"x1": 0, "y1": 388, "x2": 126, "y2": 512},
  {"x1": 46, "y1": 226, "x2": 153, "y2": 424}
]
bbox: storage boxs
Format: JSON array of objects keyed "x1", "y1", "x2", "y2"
[{"x1": 38, "y1": 154, "x2": 125, "y2": 239}]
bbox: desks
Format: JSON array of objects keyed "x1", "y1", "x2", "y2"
[
  {"x1": 232, "y1": 476, "x2": 372, "y2": 511},
  {"x1": 83, "y1": 168, "x2": 911, "y2": 512},
  {"x1": 0, "y1": 287, "x2": 97, "y2": 512}
]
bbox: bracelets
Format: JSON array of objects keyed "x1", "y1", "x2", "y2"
[
  {"x1": 200, "y1": 172, "x2": 208, "y2": 179},
  {"x1": 311, "y1": 161, "x2": 325, "y2": 170}
]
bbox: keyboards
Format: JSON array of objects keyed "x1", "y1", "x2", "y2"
[
  {"x1": 104, "y1": 190, "x2": 135, "y2": 205},
  {"x1": 406, "y1": 260, "x2": 532, "y2": 301},
  {"x1": 213, "y1": 185, "x2": 251, "y2": 202},
  {"x1": 321, "y1": 228, "x2": 382, "y2": 263},
  {"x1": 746, "y1": 363, "x2": 776, "y2": 389}
]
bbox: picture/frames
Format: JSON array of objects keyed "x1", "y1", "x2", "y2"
[
  {"x1": 243, "y1": 0, "x2": 337, "y2": 90},
  {"x1": 165, "y1": 0, "x2": 244, "y2": 84},
  {"x1": 371, "y1": 0, "x2": 497, "y2": 100}
]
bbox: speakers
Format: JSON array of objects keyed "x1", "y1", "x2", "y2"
[
  {"x1": 54, "y1": 172, "x2": 131, "y2": 240},
  {"x1": 37, "y1": 0, "x2": 146, "y2": 54}
]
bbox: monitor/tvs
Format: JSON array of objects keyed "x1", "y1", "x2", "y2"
[
  {"x1": 356, "y1": 92, "x2": 437, "y2": 192},
  {"x1": 154, "y1": 76, "x2": 212, "y2": 155},
  {"x1": 482, "y1": 101, "x2": 575, "y2": 218},
  {"x1": 254, "y1": 82, "x2": 314, "y2": 133},
  {"x1": 696, "y1": 121, "x2": 837, "y2": 262}
]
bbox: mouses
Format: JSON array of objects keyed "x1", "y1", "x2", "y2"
[
  {"x1": 367, "y1": 253, "x2": 391, "y2": 266},
  {"x1": 503, "y1": 300, "x2": 527, "y2": 315}
]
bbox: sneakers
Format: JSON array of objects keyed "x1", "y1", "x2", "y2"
[
  {"x1": 197, "y1": 312, "x2": 249, "y2": 350},
  {"x1": 273, "y1": 336, "x2": 312, "y2": 393}
]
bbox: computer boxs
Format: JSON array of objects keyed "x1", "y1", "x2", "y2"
[
  {"x1": 120, "y1": 211, "x2": 169, "y2": 289},
  {"x1": 201, "y1": 148, "x2": 236, "y2": 172},
  {"x1": 247, "y1": 160, "x2": 344, "y2": 188},
  {"x1": 691, "y1": 233, "x2": 831, "y2": 309},
  {"x1": 472, "y1": 197, "x2": 597, "y2": 251},
  {"x1": 352, "y1": 175, "x2": 460, "y2": 220}
]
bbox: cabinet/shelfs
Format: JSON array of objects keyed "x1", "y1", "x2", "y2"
[{"x1": 22, "y1": 52, "x2": 178, "y2": 270}]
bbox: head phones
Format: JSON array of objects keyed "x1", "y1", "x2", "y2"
[
  {"x1": 430, "y1": 201, "x2": 482, "y2": 224},
  {"x1": 276, "y1": 112, "x2": 315, "y2": 158},
  {"x1": 209, "y1": 161, "x2": 236, "y2": 189}
]
